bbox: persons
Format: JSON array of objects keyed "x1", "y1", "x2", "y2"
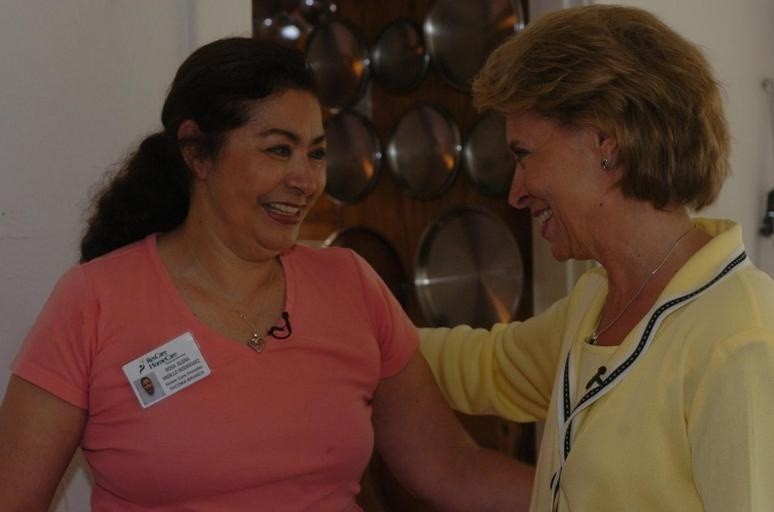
[
  {"x1": 411, "y1": 4, "x2": 773, "y2": 512},
  {"x1": 0, "y1": 36, "x2": 535, "y2": 511},
  {"x1": 138, "y1": 376, "x2": 165, "y2": 405}
]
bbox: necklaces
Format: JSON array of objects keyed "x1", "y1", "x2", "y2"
[
  {"x1": 590, "y1": 224, "x2": 698, "y2": 339},
  {"x1": 181, "y1": 233, "x2": 277, "y2": 353}
]
